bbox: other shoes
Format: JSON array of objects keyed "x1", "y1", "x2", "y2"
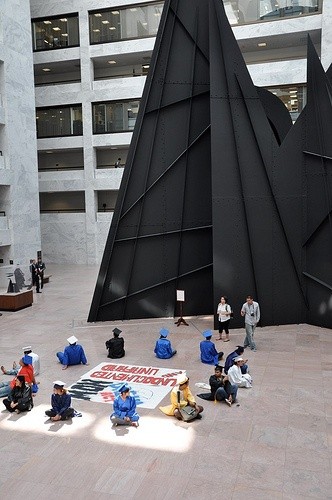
[
  {"x1": 215, "y1": 336, "x2": 229, "y2": 342},
  {"x1": 15, "y1": 409, "x2": 20, "y2": 414},
  {"x1": 132, "y1": 421, "x2": 139, "y2": 427},
  {"x1": 51, "y1": 416, "x2": 61, "y2": 421},
  {"x1": 1, "y1": 366, "x2": 7, "y2": 374},
  {"x1": 13, "y1": 361, "x2": 17, "y2": 369},
  {"x1": 28, "y1": 285, "x2": 43, "y2": 293},
  {"x1": 244, "y1": 344, "x2": 256, "y2": 352},
  {"x1": 225, "y1": 397, "x2": 234, "y2": 406}
]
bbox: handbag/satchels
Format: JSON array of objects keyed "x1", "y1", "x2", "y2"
[{"x1": 179, "y1": 405, "x2": 199, "y2": 422}]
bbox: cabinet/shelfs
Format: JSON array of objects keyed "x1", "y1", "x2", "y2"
[{"x1": 0, "y1": 264, "x2": 34, "y2": 312}]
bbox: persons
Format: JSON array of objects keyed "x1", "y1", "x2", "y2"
[
  {"x1": 1, "y1": 346, "x2": 41, "y2": 376},
  {"x1": 0, "y1": 354, "x2": 40, "y2": 399},
  {"x1": 3, "y1": 375, "x2": 34, "y2": 414},
  {"x1": 217, "y1": 296, "x2": 232, "y2": 342},
  {"x1": 109, "y1": 384, "x2": 140, "y2": 427},
  {"x1": 105, "y1": 328, "x2": 125, "y2": 359},
  {"x1": 44, "y1": 381, "x2": 82, "y2": 422},
  {"x1": 57, "y1": 336, "x2": 89, "y2": 370},
  {"x1": 199, "y1": 330, "x2": 224, "y2": 365},
  {"x1": 13, "y1": 257, "x2": 46, "y2": 293},
  {"x1": 196, "y1": 364, "x2": 237, "y2": 407},
  {"x1": 158, "y1": 372, "x2": 203, "y2": 421},
  {"x1": 224, "y1": 346, "x2": 252, "y2": 388},
  {"x1": 241, "y1": 296, "x2": 260, "y2": 351},
  {"x1": 154, "y1": 328, "x2": 177, "y2": 360}
]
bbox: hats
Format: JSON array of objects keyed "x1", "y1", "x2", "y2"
[
  {"x1": 53, "y1": 381, "x2": 66, "y2": 389},
  {"x1": 160, "y1": 329, "x2": 169, "y2": 337},
  {"x1": 22, "y1": 345, "x2": 31, "y2": 351},
  {"x1": 233, "y1": 355, "x2": 244, "y2": 362},
  {"x1": 202, "y1": 329, "x2": 213, "y2": 338},
  {"x1": 237, "y1": 346, "x2": 245, "y2": 350},
  {"x1": 215, "y1": 364, "x2": 225, "y2": 371},
  {"x1": 22, "y1": 355, "x2": 32, "y2": 364},
  {"x1": 117, "y1": 384, "x2": 129, "y2": 392},
  {"x1": 66, "y1": 336, "x2": 78, "y2": 344},
  {"x1": 179, "y1": 376, "x2": 188, "y2": 385},
  {"x1": 112, "y1": 328, "x2": 122, "y2": 335}
]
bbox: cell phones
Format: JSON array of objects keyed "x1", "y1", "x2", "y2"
[{"x1": 241, "y1": 310, "x2": 245, "y2": 314}]
